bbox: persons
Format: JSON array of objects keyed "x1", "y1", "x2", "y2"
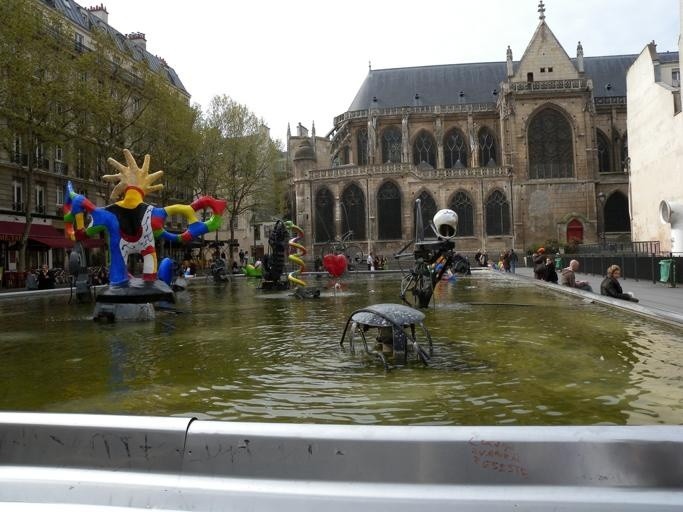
[
  {"x1": 479, "y1": 249, "x2": 488, "y2": 267},
  {"x1": 243, "y1": 251, "x2": 248, "y2": 264},
  {"x1": 187, "y1": 262, "x2": 196, "y2": 276},
  {"x1": 24, "y1": 267, "x2": 56, "y2": 292},
  {"x1": 541, "y1": 256, "x2": 558, "y2": 283},
  {"x1": 231, "y1": 262, "x2": 241, "y2": 273},
  {"x1": 313, "y1": 256, "x2": 321, "y2": 272},
  {"x1": 600, "y1": 264, "x2": 639, "y2": 303},
  {"x1": 501, "y1": 248, "x2": 511, "y2": 273},
  {"x1": 373, "y1": 257, "x2": 379, "y2": 270},
  {"x1": 474, "y1": 249, "x2": 481, "y2": 265},
  {"x1": 531, "y1": 247, "x2": 547, "y2": 281},
  {"x1": 366, "y1": 253, "x2": 372, "y2": 270},
  {"x1": 238, "y1": 249, "x2": 244, "y2": 265},
  {"x1": 508, "y1": 249, "x2": 519, "y2": 274},
  {"x1": 254, "y1": 255, "x2": 262, "y2": 268},
  {"x1": 561, "y1": 259, "x2": 594, "y2": 293}
]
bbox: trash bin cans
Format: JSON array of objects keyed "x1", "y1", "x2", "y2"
[
  {"x1": 658, "y1": 259, "x2": 677, "y2": 288},
  {"x1": 554, "y1": 257, "x2": 563, "y2": 270}
]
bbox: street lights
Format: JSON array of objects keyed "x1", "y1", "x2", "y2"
[
  {"x1": 250, "y1": 207, "x2": 257, "y2": 252},
  {"x1": 363, "y1": 173, "x2": 372, "y2": 231},
  {"x1": 477, "y1": 176, "x2": 485, "y2": 250},
  {"x1": 595, "y1": 192, "x2": 607, "y2": 236},
  {"x1": 286, "y1": 180, "x2": 295, "y2": 225},
  {"x1": 501, "y1": 164, "x2": 516, "y2": 249},
  {"x1": 306, "y1": 178, "x2": 315, "y2": 239}
]
